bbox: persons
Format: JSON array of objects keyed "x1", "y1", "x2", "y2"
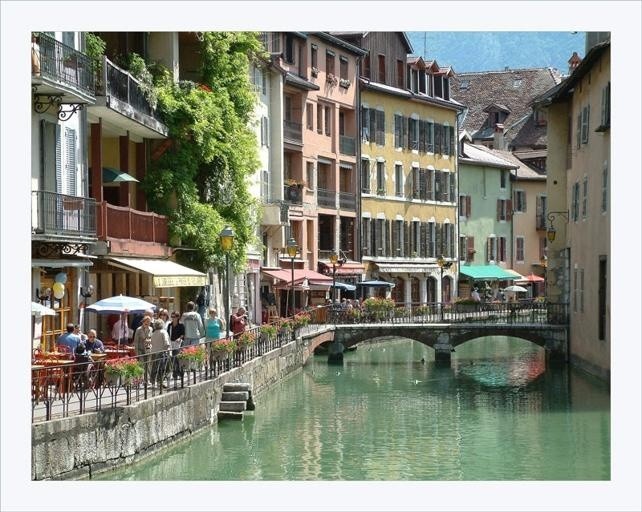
[
  {"x1": 470, "y1": 286, "x2": 481, "y2": 302},
  {"x1": 231, "y1": 306, "x2": 250, "y2": 361},
  {"x1": 165, "y1": 309, "x2": 186, "y2": 380},
  {"x1": 156, "y1": 306, "x2": 173, "y2": 381},
  {"x1": 72, "y1": 324, "x2": 88, "y2": 342},
  {"x1": 180, "y1": 300, "x2": 206, "y2": 372},
  {"x1": 132, "y1": 315, "x2": 154, "y2": 385},
  {"x1": 54, "y1": 322, "x2": 82, "y2": 393},
  {"x1": 324, "y1": 294, "x2": 376, "y2": 324},
  {"x1": 202, "y1": 306, "x2": 224, "y2": 370},
  {"x1": 495, "y1": 287, "x2": 506, "y2": 301},
  {"x1": 149, "y1": 318, "x2": 172, "y2": 389},
  {"x1": 131, "y1": 308, "x2": 157, "y2": 347},
  {"x1": 80, "y1": 328, "x2": 106, "y2": 390},
  {"x1": 109, "y1": 311, "x2": 130, "y2": 346}
]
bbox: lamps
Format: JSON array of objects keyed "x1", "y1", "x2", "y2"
[{"x1": 547, "y1": 207, "x2": 569, "y2": 244}]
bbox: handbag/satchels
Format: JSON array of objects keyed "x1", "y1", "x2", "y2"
[
  {"x1": 171, "y1": 340, "x2": 182, "y2": 349},
  {"x1": 230, "y1": 315, "x2": 234, "y2": 331}
]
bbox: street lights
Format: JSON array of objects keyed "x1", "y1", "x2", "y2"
[
  {"x1": 541, "y1": 253, "x2": 547, "y2": 297},
  {"x1": 218, "y1": 224, "x2": 235, "y2": 370},
  {"x1": 436, "y1": 253, "x2": 445, "y2": 322},
  {"x1": 329, "y1": 248, "x2": 338, "y2": 304},
  {"x1": 286, "y1": 237, "x2": 298, "y2": 340}
]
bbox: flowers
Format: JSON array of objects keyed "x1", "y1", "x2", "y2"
[{"x1": 175, "y1": 312, "x2": 310, "y2": 370}]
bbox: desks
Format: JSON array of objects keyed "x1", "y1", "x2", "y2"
[{"x1": 31, "y1": 336, "x2": 138, "y2": 403}]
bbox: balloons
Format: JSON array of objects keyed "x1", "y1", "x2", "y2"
[
  {"x1": 53, "y1": 291, "x2": 65, "y2": 299},
  {"x1": 53, "y1": 282, "x2": 65, "y2": 293},
  {"x1": 54, "y1": 272, "x2": 68, "y2": 284}
]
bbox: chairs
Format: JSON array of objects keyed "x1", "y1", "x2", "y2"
[{"x1": 267, "y1": 307, "x2": 279, "y2": 325}]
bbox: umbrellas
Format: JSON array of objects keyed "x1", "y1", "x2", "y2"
[
  {"x1": 502, "y1": 284, "x2": 528, "y2": 301},
  {"x1": 85, "y1": 292, "x2": 156, "y2": 355},
  {"x1": 32, "y1": 300, "x2": 56, "y2": 316},
  {"x1": 87, "y1": 166, "x2": 141, "y2": 185}
]
